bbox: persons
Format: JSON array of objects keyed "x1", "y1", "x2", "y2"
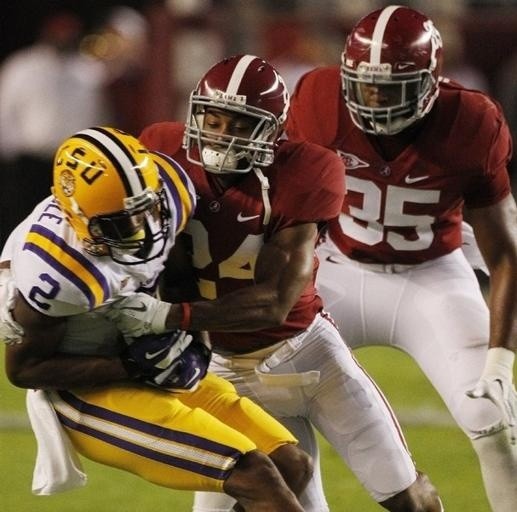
[
  {"x1": 0, "y1": 52, "x2": 445, "y2": 512},
  {"x1": 5, "y1": 125, "x2": 316, "y2": 512},
  {"x1": 192, "y1": 4, "x2": 517, "y2": 512},
  {"x1": 2, "y1": 2, "x2": 517, "y2": 255}
]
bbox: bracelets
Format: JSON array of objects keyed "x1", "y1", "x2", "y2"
[{"x1": 182, "y1": 303, "x2": 191, "y2": 331}]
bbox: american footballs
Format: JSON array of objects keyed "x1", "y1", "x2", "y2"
[{"x1": 128, "y1": 330, "x2": 206, "y2": 389}]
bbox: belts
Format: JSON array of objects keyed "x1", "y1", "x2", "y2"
[
  {"x1": 213, "y1": 312, "x2": 321, "y2": 386},
  {"x1": 348, "y1": 259, "x2": 419, "y2": 274}
]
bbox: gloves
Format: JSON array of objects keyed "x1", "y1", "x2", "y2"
[
  {"x1": 123, "y1": 327, "x2": 212, "y2": 395},
  {"x1": 107, "y1": 292, "x2": 172, "y2": 343},
  {"x1": 0, "y1": 269, "x2": 25, "y2": 348},
  {"x1": 466, "y1": 347, "x2": 517, "y2": 449}
]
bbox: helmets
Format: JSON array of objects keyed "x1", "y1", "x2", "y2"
[
  {"x1": 54, "y1": 125, "x2": 172, "y2": 265},
  {"x1": 181, "y1": 55, "x2": 291, "y2": 176},
  {"x1": 338, "y1": 5, "x2": 443, "y2": 138}
]
